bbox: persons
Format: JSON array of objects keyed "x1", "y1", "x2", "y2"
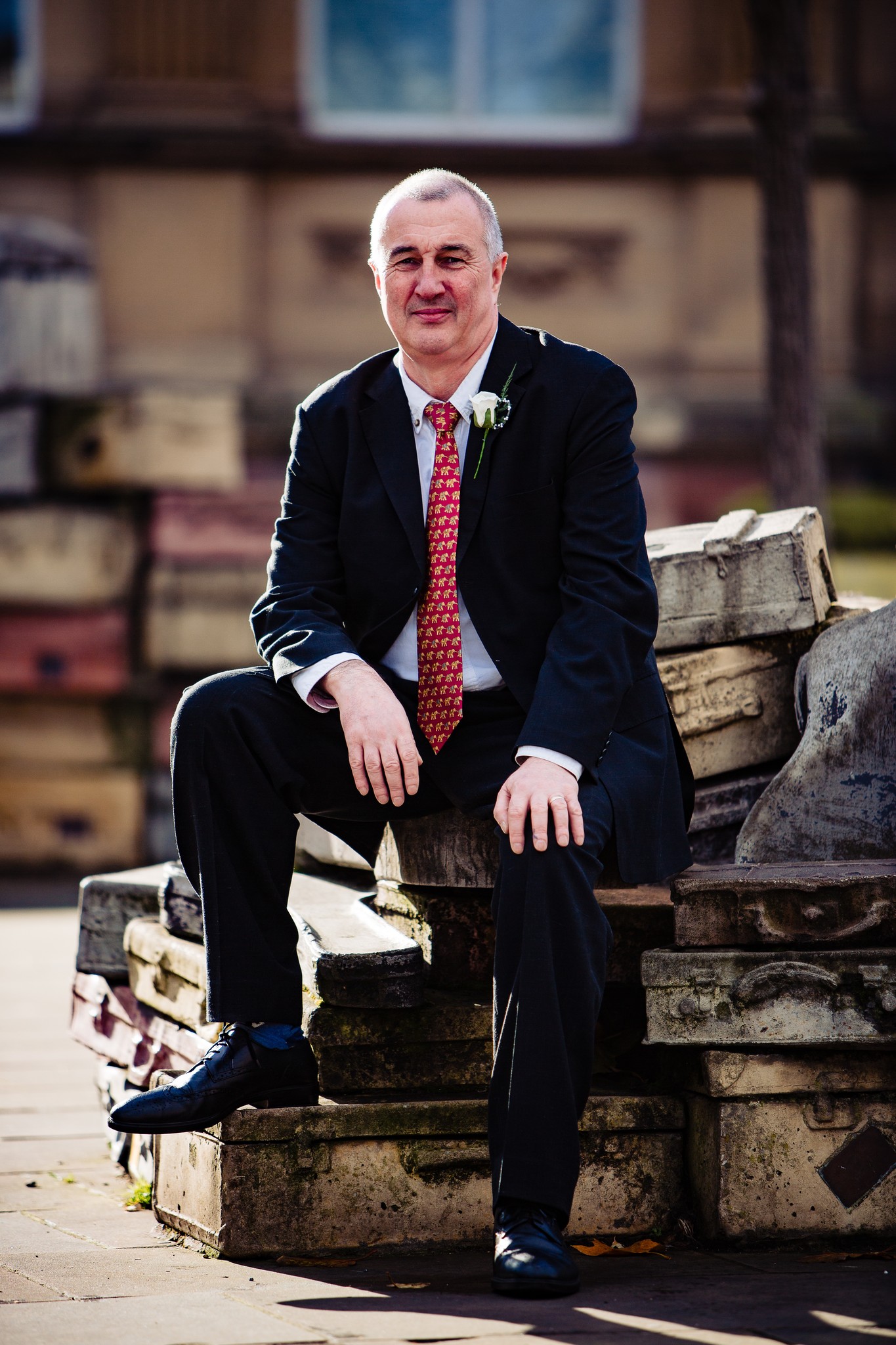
[{"x1": 99, "y1": 169, "x2": 702, "y2": 1306}]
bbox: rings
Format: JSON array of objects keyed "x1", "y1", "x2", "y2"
[{"x1": 548, "y1": 795, "x2": 566, "y2": 805}]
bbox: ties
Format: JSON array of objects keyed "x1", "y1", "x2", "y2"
[{"x1": 416, "y1": 396, "x2": 468, "y2": 755}]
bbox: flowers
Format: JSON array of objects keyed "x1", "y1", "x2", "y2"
[{"x1": 466, "y1": 390, "x2": 509, "y2": 480}]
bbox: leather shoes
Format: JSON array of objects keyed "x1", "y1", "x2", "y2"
[
  {"x1": 489, "y1": 1211, "x2": 587, "y2": 1302},
  {"x1": 103, "y1": 1006, "x2": 319, "y2": 1137}
]
